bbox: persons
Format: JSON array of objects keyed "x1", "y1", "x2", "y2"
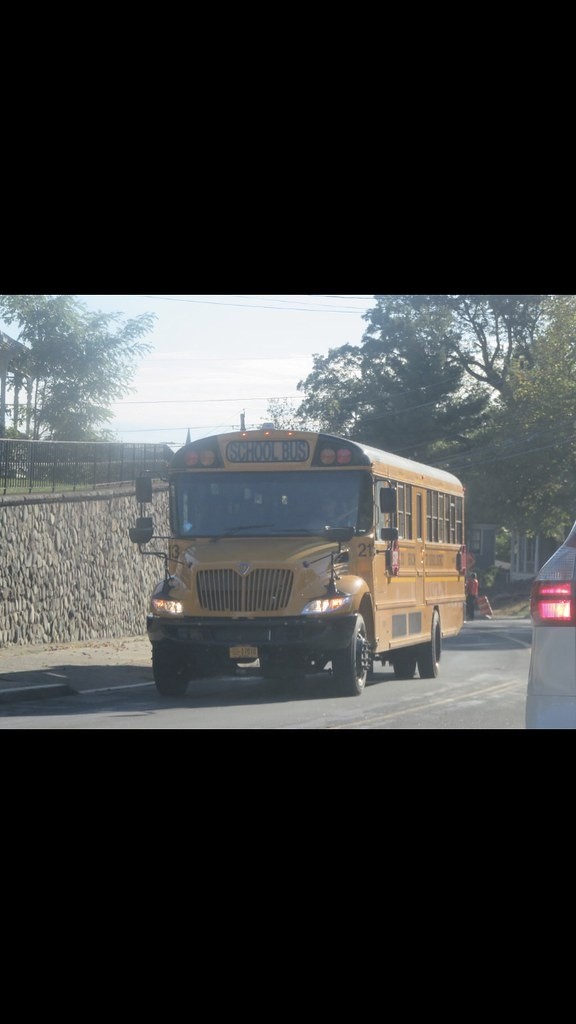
[
  {"x1": 305, "y1": 496, "x2": 352, "y2": 529},
  {"x1": 464, "y1": 572, "x2": 478, "y2": 620}
]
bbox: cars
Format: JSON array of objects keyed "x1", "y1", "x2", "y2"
[{"x1": 523, "y1": 519, "x2": 576, "y2": 728}]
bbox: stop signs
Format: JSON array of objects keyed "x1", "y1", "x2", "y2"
[{"x1": 390, "y1": 548, "x2": 399, "y2": 574}]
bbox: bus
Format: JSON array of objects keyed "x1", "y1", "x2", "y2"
[{"x1": 129, "y1": 428, "x2": 465, "y2": 695}]
